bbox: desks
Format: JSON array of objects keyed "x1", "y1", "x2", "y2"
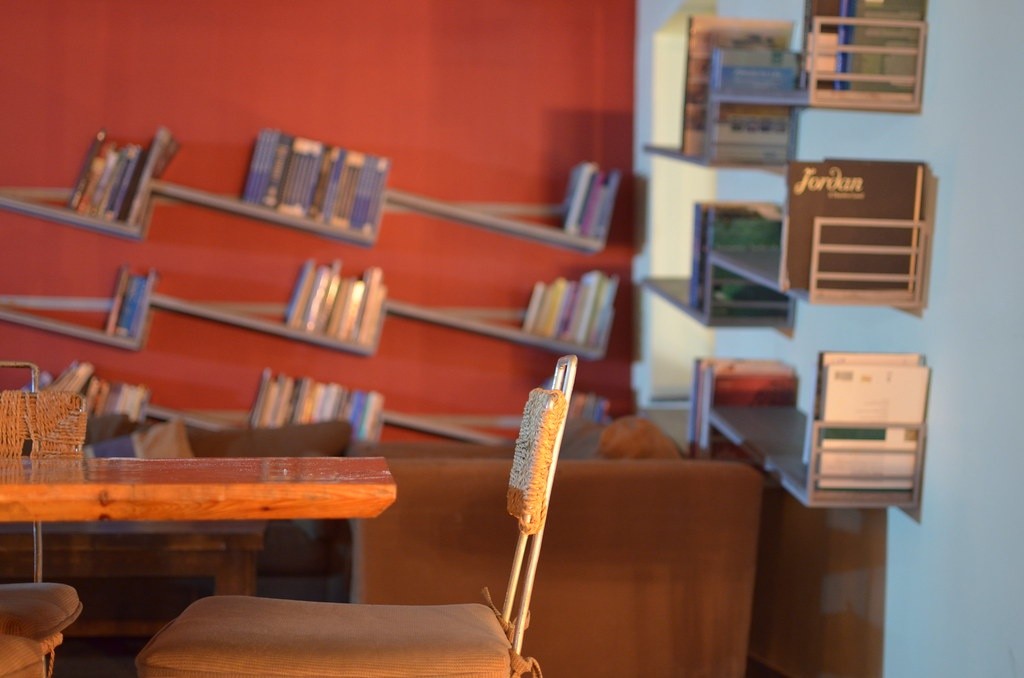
[{"x1": 0, "y1": 454, "x2": 398, "y2": 524}]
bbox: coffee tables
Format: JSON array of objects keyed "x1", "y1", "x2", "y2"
[{"x1": 0, "y1": 534, "x2": 267, "y2": 635}]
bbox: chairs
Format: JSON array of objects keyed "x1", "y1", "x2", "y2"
[
  {"x1": 0, "y1": 389, "x2": 89, "y2": 632},
  {"x1": 120, "y1": 351, "x2": 579, "y2": 678}
]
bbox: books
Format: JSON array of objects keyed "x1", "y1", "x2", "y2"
[
  {"x1": 564, "y1": 162, "x2": 624, "y2": 248},
  {"x1": 291, "y1": 259, "x2": 390, "y2": 348},
  {"x1": 253, "y1": 367, "x2": 387, "y2": 445},
  {"x1": 689, "y1": 202, "x2": 792, "y2": 322},
  {"x1": 682, "y1": 16, "x2": 803, "y2": 168},
  {"x1": 800, "y1": 0, "x2": 927, "y2": 91},
  {"x1": 106, "y1": 267, "x2": 159, "y2": 338},
  {"x1": 800, "y1": 350, "x2": 932, "y2": 502},
  {"x1": 69, "y1": 127, "x2": 181, "y2": 225},
  {"x1": 698, "y1": 358, "x2": 797, "y2": 410},
  {"x1": 17, "y1": 361, "x2": 146, "y2": 425},
  {"x1": 243, "y1": 127, "x2": 389, "y2": 234},
  {"x1": 779, "y1": 156, "x2": 934, "y2": 288},
  {"x1": 523, "y1": 272, "x2": 620, "y2": 352}
]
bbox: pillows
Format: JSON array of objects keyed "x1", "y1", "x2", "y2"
[{"x1": 84, "y1": 419, "x2": 201, "y2": 458}]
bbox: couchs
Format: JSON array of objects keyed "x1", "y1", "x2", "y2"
[
  {"x1": 0, "y1": 411, "x2": 354, "y2": 595},
  {"x1": 352, "y1": 417, "x2": 764, "y2": 678}
]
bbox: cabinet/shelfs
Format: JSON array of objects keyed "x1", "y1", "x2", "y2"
[{"x1": 0, "y1": 1, "x2": 936, "y2": 525}]
